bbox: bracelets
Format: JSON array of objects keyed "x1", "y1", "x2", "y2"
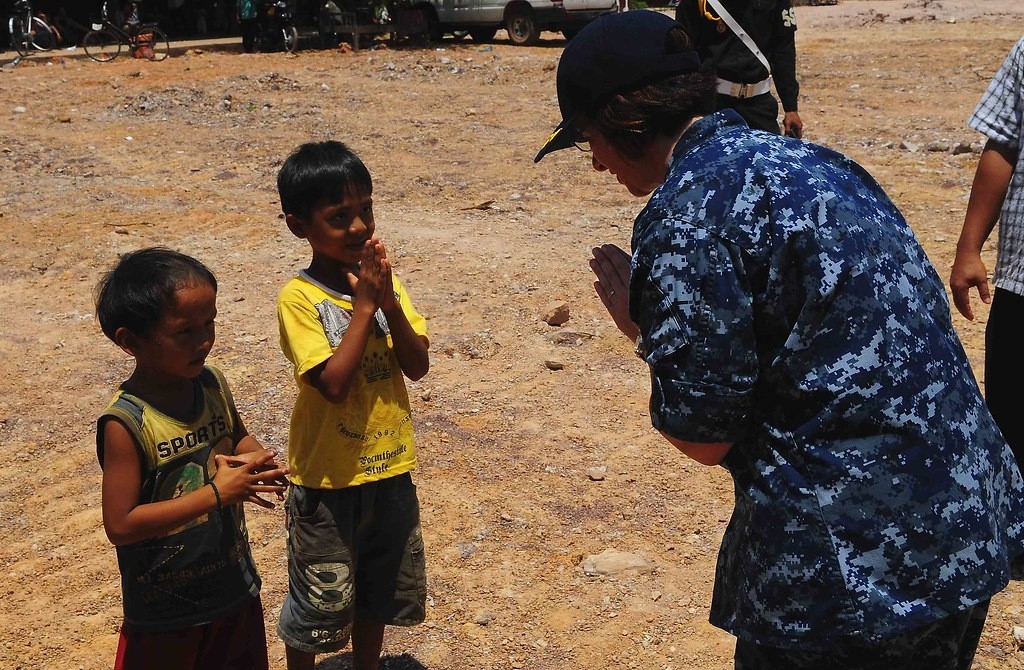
[{"x1": 204, "y1": 482, "x2": 222, "y2": 514}]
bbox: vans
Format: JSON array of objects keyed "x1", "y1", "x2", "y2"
[{"x1": 403, "y1": 0, "x2": 629, "y2": 46}]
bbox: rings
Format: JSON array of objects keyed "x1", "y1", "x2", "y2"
[{"x1": 607, "y1": 290, "x2": 615, "y2": 300}]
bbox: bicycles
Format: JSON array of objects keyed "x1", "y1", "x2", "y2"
[
  {"x1": 85, "y1": 1, "x2": 170, "y2": 61},
  {"x1": 9, "y1": 0, "x2": 55, "y2": 56}
]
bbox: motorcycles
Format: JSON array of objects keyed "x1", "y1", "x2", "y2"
[{"x1": 250, "y1": 1, "x2": 298, "y2": 53}]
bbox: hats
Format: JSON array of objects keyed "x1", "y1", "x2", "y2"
[{"x1": 533, "y1": 10, "x2": 700, "y2": 165}]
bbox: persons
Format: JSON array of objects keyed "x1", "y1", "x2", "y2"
[
  {"x1": 948, "y1": 30, "x2": 1024, "y2": 578},
  {"x1": 673, "y1": 0, "x2": 804, "y2": 142},
  {"x1": 555, "y1": 7, "x2": 1024, "y2": 667},
  {"x1": 95, "y1": 247, "x2": 291, "y2": 670},
  {"x1": 0, "y1": 0, "x2": 412, "y2": 54},
  {"x1": 275, "y1": 140, "x2": 430, "y2": 670}
]
